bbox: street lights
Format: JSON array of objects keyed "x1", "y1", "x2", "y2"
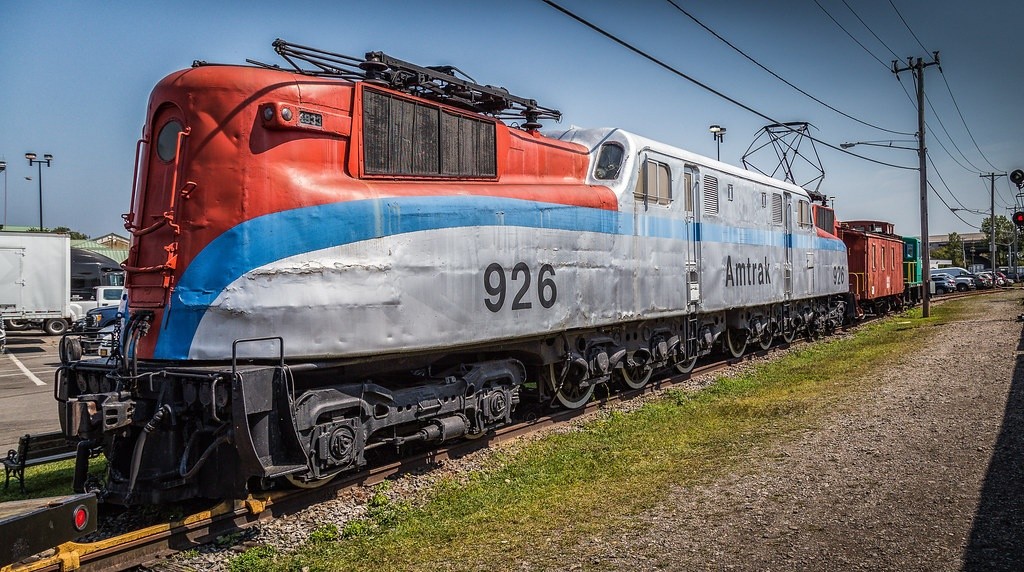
[
  {"x1": 25, "y1": 152, "x2": 52, "y2": 231},
  {"x1": 710, "y1": 124, "x2": 727, "y2": 161},
  {"x1": 950, "y1": 207, "x2": 998, "y2": 287},
  {"x1": 839, "y1": 137, "x2": 931, "y2": 318}
]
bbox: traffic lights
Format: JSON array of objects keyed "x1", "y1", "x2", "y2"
[
  {"x1": 1010, "y1": 168, "x2": 1024, "y2": 183},
  {"x1": 1013, "y1": 212, "x2": 1024, "y2": 226}
]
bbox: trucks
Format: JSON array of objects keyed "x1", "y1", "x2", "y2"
[{"x1": 0, "y1": 229, "x2": 125, "y2": 334}]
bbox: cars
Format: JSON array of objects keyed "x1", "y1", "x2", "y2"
[
  {"x1": 930, "y1": 266, "x2": 1023, "y2": 295},
  {"x1": 71, "y1": 305, "x2": 120, "y2": 336}
]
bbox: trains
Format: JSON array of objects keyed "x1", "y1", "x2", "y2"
[{"x1": 0, "y1": 35, "x2": 923, "y2": 567}]
